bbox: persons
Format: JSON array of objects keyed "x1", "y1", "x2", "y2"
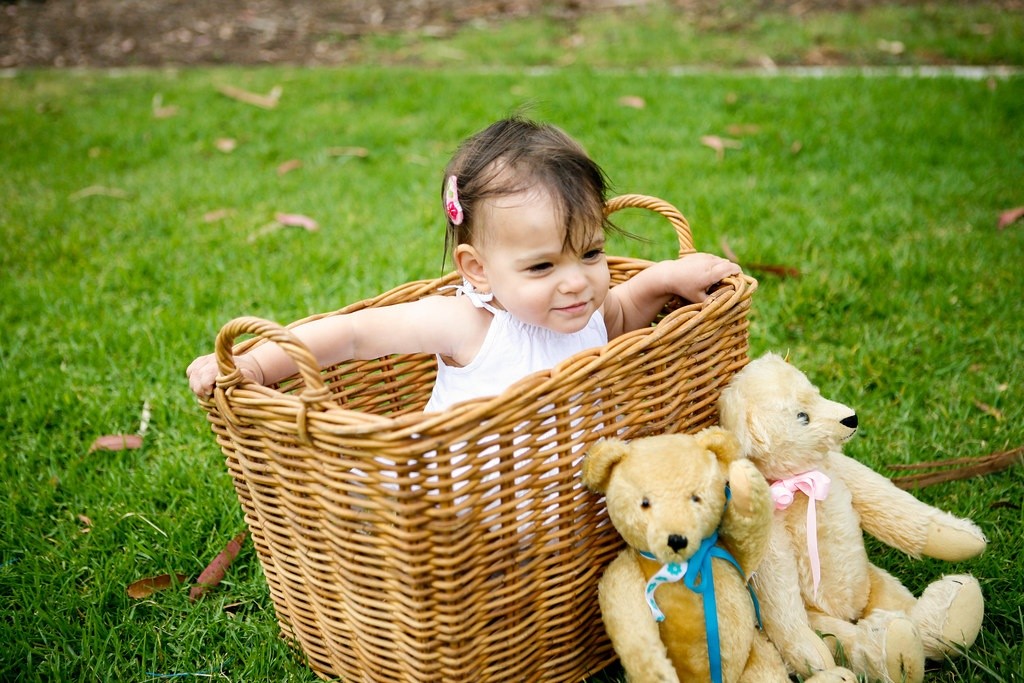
[{"x1": 185, "y1": 115, "x2": 742, "y2": 542}]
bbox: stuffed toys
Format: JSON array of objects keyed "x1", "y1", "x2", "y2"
[
  {"x1": 583, "y1": 425, "x2": 856, "y2": 683},
  {"x1": 718, "y1": 351, "x2": 986, "y2": 683}
]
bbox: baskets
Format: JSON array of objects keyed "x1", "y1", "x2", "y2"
[{"x1": 193, "y1": 192, "x2": 759, "y2": 683}]
